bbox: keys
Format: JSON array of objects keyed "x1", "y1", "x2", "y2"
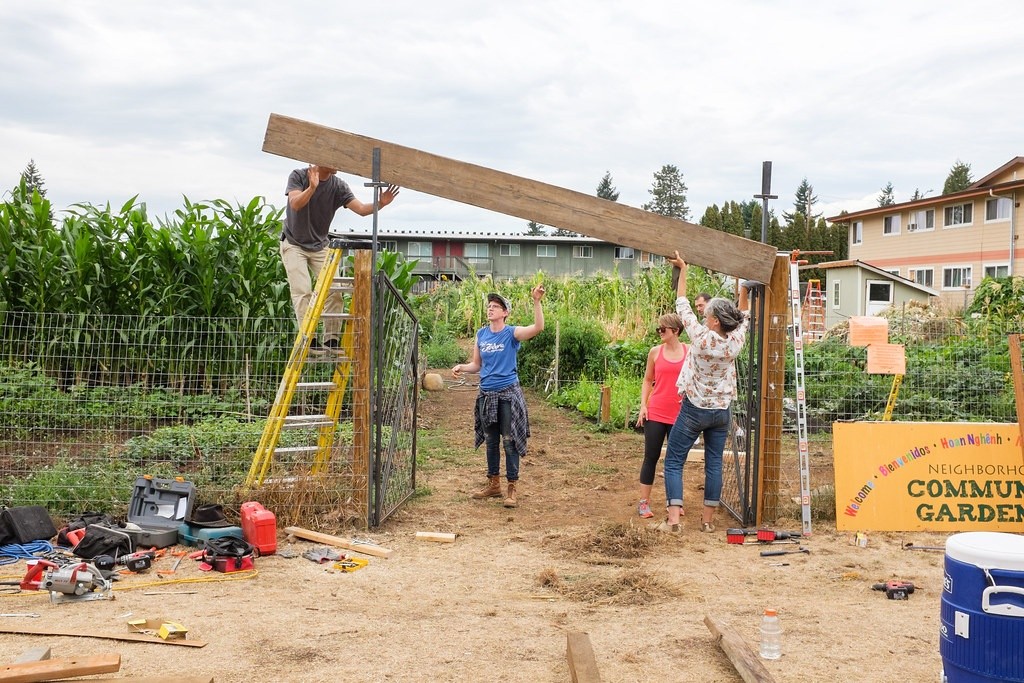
[{"x1": 280, "y1": 233, "x2": 285, "y2": 241}]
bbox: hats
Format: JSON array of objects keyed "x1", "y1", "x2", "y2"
[{"x1": 486, "y1": 293, "x2": 511, "y2": 312}]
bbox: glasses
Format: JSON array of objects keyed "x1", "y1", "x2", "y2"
[
  {"x1": 485, "y1": 304, "x2": 505, "y2": 310},
  {"x1": 656, "y1": 326, "x2": 677, "y2": 333}
]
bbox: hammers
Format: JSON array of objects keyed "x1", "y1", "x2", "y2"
[{"x1": 758, "y1": 545, "x2": 810, "y2": 556}]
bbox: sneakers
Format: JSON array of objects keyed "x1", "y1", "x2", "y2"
[
  {"x1": 309, "y1": 338, "x2": 325, "y2": 357},
  {"x1": 699, "y1": 519, "x2": 716, "y2": 532},
  {"x1": 637, "y1": 499, "x2": 652, "y2": 518},
  {"x1": 647, "y1": 519, "x2": 682, "y2": 536},
  {"x1": 323, "y1": 338, "x2": 345, "y2": 354},
  {"x1": 666, "y1": 506, "x2": 684, "y2": 515}
]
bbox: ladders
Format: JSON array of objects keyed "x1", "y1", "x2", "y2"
[
  {"x1": 240, "y1": 236, "x2": 381, "y2": 498},
  {"x1": 796, "y1": 278, "x2": 824, "y2": 347}
]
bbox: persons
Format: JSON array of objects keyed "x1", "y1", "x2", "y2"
[
  {"x1": 279, "y1": 162, "x2": 400, "y2": 355},
  {"x1": 452, "y1": 285, "x2": 546, "y2": 506},
  {"x1": 647, "y1": 250, "x2": 766, "y2": 534},
  {"x1": 693, "y1": 294, "x2": 745, "y2": 446},
  {"x1": 635, "y1": 314, "x2": 692, "y2": 518}
]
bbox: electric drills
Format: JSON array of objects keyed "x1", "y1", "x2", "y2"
[
  {"x1": 727, "y1": 529, "x2": 758, "y2": 544},
  {"x1": 16, "y1": 559, "x2": 108, "y2": 597},
  {"x1": 115, "y1": 550, "x2": 155, "y2": 572},
  {"x1": 868, "y1": 580, "x2": 915, "y2": 601},
  {"x1": 757, "y1": 530, "x2": 797, "y2": 542}
]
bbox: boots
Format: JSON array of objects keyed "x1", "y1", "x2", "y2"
[
  {"x1": 503, "y1": 480, "x2": 517, "y2": 507},
  {"x1": 473, "y1": 476, "x2": 502, "y2": 499}
]
